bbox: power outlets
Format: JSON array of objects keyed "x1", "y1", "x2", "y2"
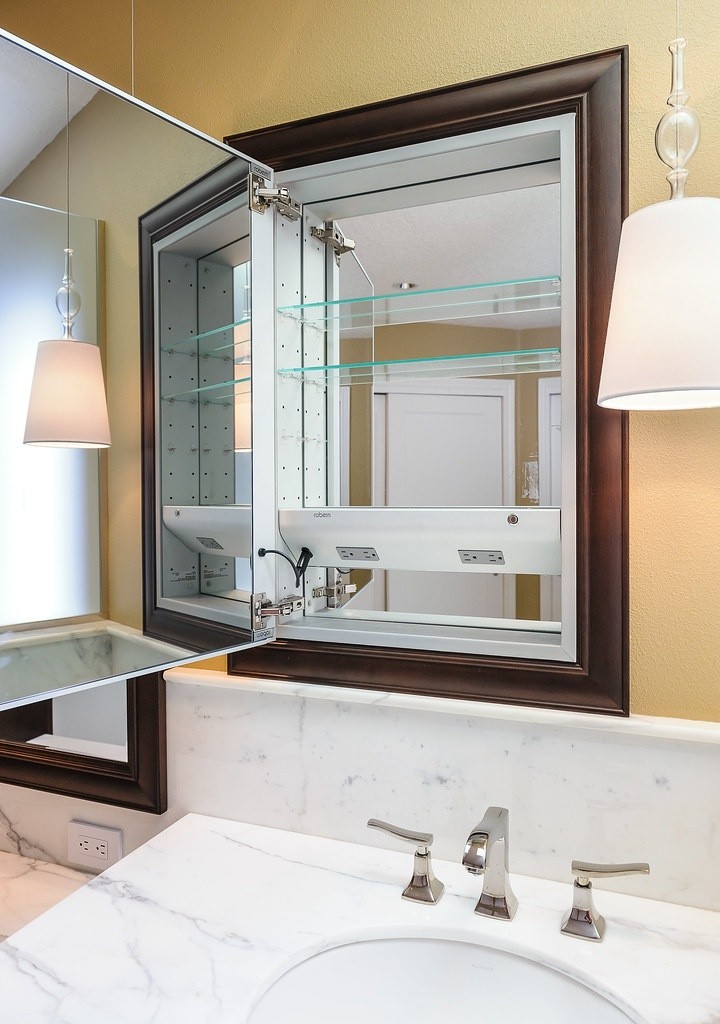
[{"x1": 66, "y1": 819, "x2": 124, "y2": 870}]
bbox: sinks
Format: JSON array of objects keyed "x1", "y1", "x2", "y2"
[{"x1": 242, "y1": 926, "x2": 651, "y2": 1023}]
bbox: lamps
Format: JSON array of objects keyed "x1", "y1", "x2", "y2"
[
  {"x1": 590, "y1": 37, "x2": 719, "y2": 414},
  {"x1": 18, "y1": 71, "x2": 112, "y2": 452}
]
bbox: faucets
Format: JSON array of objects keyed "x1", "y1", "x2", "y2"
[{"x1": 461, "y1": 806, "x2": 520, "y2": 921}]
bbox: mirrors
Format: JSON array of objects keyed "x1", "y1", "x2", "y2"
[
  {"x1": 0, "y1": 665, "x2": 171, "y2": 815},
  {"x1": 293, "y1": 155, "x2": 563, "y2": 633},
  {"x1": 0, "y1": 27, "x2": 256, "y2": 718}
]
